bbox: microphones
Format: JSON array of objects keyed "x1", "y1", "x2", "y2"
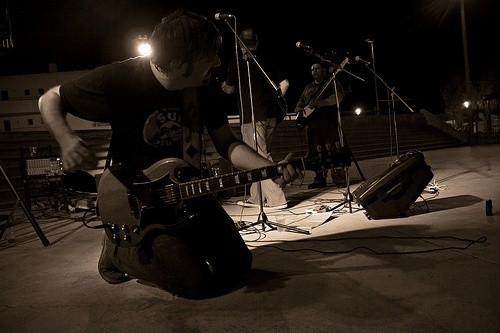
[
  {"x1": 296, "y1": 42, "x2": 311, "y2": 49},
  {"x1": 355, "y1": 56, "x2": 371, "y2": 64},
  {"x1": 215, "y1": 13, "x2": 234, "y2": 20}
]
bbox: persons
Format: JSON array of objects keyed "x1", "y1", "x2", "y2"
[
  {"x1": 37, "y1": 11, "x2": 304, "y2": 299},
  {"x1": 295, "y1": 62, "x2": 347, "y2": 189},
  {"x1": 221, "y1": 29, "x2": 290, "y2": 209}
]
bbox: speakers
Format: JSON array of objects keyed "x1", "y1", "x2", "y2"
[{"x1": 352, "y1": 150, "x2": 433, "y2": 220}]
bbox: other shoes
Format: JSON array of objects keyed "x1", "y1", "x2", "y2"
[
  {"x1": 307, "y1": 174, "x2": 328, "y2": 189},
  {"x1": 97, "y1": 229, "x2": 129, "y2": 283}
]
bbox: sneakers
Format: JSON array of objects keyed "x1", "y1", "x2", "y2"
[{"x1": 237, "y1": 199, "x2": 289, "y2": 210}]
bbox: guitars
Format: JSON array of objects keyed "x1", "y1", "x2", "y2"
[
  {"x1": 296, "y1": 57, "x2": 350, "y2": 131},
  {"x1": 97, "y1": 141, "x2": 352, "y2": 248}
]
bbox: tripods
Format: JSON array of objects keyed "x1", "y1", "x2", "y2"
[
  {"x1": 225, "y1": 19, "x2": 309, "y2": 234},
  {"x1": 303, "y1": 48, "x2": 366, "y2": 214}
]
